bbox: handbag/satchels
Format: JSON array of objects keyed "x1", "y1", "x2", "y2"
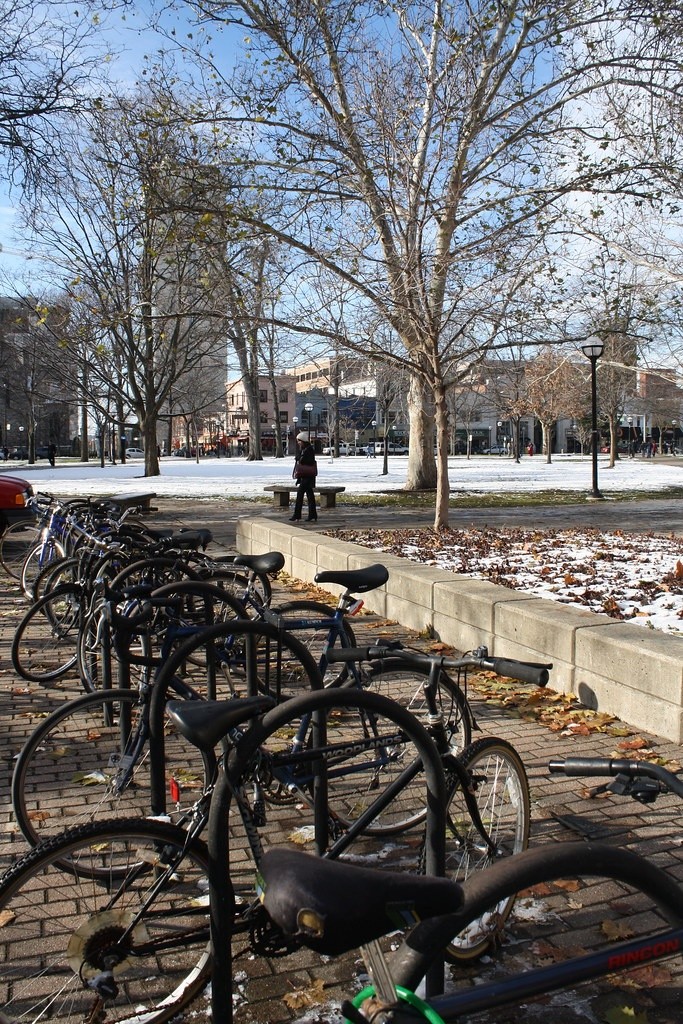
[{"x1": 293, "y1": 447, "x2": 317, "y2": 477}]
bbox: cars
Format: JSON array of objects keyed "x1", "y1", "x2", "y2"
[
  {"x1": 0, "y1": 443, "x2": 74, "y2": 461},
  {"x1": 125, "y1": 447, "x2": 145, "y2": 459},
  {"x1": 482, "y1": 444, "x2": 510, "y2": 455},
  {"x1": 600, "y1": 441, "x2": 683, "y2": 455},
  {"x1": 322, "y1": 441, "x2": 409, "y2": 456},
  {"x1": 0, "y1": 474, "x2": 39, "y2": 538}
]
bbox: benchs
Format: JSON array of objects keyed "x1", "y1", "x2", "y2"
[
  {"x1": 263, "y1": 484, "x2": 345, "y2": 509},
  {"x1": 109, "y1": 492, "x2": 157, "y2": 516}
]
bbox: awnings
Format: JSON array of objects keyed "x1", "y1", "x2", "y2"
[
  {"x1": 387, "y1": 423, "x2": 410, "y2": 431},
  {"x1": 225, "y1": 432, "x2": 238, "y2": 437},
  {"x1": 456, "y1": 421, "x2": 490, "y2": 430}
]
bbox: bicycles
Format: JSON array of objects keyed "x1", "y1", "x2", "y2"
[
  {"x1": 0, "y1": 485, "x2": 553, "y2": 1024},
  {"x1": 251, "y1": 757, "x2": 683, "y2": 1023}
]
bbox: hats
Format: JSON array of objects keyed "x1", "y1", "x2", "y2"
[{"x1": 296, "y1": 432, "x2": 308, "y2": 442}]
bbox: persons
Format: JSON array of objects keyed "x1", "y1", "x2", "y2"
[
  {"x1": 366, "y1": 444, "x2": 372, "y2": 458},
  {"x1": 627, "y1": 438, "x2": 674, "y2": 458},
  {"x1": 211, "y1": 447, "x2": 217, "y2": 455},
  {"x1": 288, "y1": 431, "x2": 319, "y2": 522},
  {"x1": 2, "y1": 445, "x2": 9, "y2": 460},
  {"x1": 527, "y1": 442, "x2": 533, "y2": 456},
  {"x1": 47, "y1": 439, "x2": 55, "y2": 467},
  {"x1": 241, "y1": 446, "x2": 245, "y2": 457},
  {"x1": 201, "y1": 445, "x2": 205, "y2": 455}
]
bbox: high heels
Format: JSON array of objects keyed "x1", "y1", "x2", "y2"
[
  {"x1": 305, "y1": 515, "x2": 317, "y2": 521},
  {"x1": 289, "y1": 515, "x2": 300, "y2": 522}
]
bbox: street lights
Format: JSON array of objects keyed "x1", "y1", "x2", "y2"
[
  {"x1": 489, "y1": 426, "x2": 493, "y2": 455},
  {"x1": 580, "y1": 335, "x2": 605, "y2": 498},
  {"x1": 392, "y1": 425, "x2": 396, "y2": 456},
  {"x1": 216, "y1": 420, "x2": 221, "y2": 458},
  {"x1": 305, "y1": 402, "x2": 314, "y2": 444},
  {"x1": 110, "y1": 408, "x2": 117, "y2": 465},
  {"x1": 18, "y1": 425, "x2": 24, "y2": 460},
  {"x1": 672, "y1": 419, "x2": 677, "y2": 454},
  {"x1": 627, "y1": 417, "x2": 633, "y2": 458},
  {"x1": 271, "y1": 424, "x2": 276, "y2": 457},
  {"x1": 371, "y1": 421, "x2": 378, "y2": 457},
  {"x1": 522, "y1": 426, "x2": 526, "y2": 454},
  {"x1": 497, "y1": 421, "x2": 502, "y2": 457},
  {"x1": 292, "y1": 417, "x2": 298, "y2": 456}
]
